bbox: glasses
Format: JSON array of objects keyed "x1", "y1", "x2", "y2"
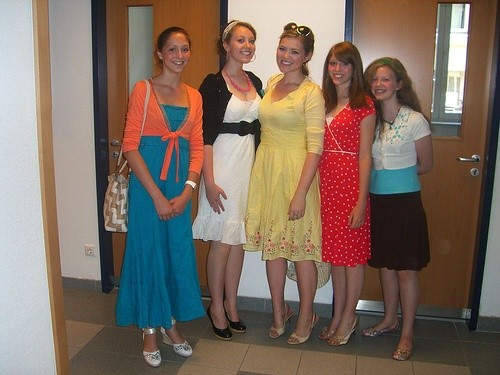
[{"x1": 284, "y1": 22, "x2": 312, "y2": 36}]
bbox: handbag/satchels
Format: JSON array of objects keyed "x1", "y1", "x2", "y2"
[
  {"x1": 286, "y1": 261, "x2": 331, "y2": 289},
  {"x1": 103, "y1": 173, "x2": 130, "y2": 233}
]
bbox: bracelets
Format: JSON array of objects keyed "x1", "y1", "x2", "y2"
[{"x1": 184, "y1": 179, "x2": 197, "y2": 188}]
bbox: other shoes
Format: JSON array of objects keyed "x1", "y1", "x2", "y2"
[
  {"x1": 362, "y1": 320, "x2": 400, "y2": 337},
  {"x1": 392, "y1": 333, "x2": 416, "y2": 361}
]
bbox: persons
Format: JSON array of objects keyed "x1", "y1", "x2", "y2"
[
  {"x1": 362, "y1": 58, "x2": 434, "y2": 360},
  {"x1": 319, "y1": 40, "x2": 376, "y2": 343},
  {"x1": 196, "y1": 19, "x2": 265, "y2": 337},
  {"x1": 246, "y1": 23, "x2": 323, "y2": 345},
  {"x1": 123, "y1": 25, "x2": 205, "y2": 364}
]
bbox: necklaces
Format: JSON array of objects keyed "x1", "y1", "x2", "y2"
[{"x1": 225, "y1": 67, "x2": 253, "y2": 92}]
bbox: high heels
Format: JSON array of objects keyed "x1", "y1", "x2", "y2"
[
  {"x1": 327, "y1": 314, "x2": 359, "y2": 346},
  {"x1": 223, "y1": 301, "x2": 247, "y2": 332},
  {"x1": 287, "y1": 312, "x2": 318, "y2": 344},
  {"x1": 142, "y1": 328, "x2": 161, "y2": 368},
  {"x1": 160, "y1": 318, "x2": 193, "y2": 357},
  {"x1": 319, "y1": 313, "x2": 343, "y2": 339},
  {"x1": 269, "y1": 301, "x2": 294, "y2": 338},
  {"x1": 207, "y1": 304, "x2": 232, "y2": 340}
]
